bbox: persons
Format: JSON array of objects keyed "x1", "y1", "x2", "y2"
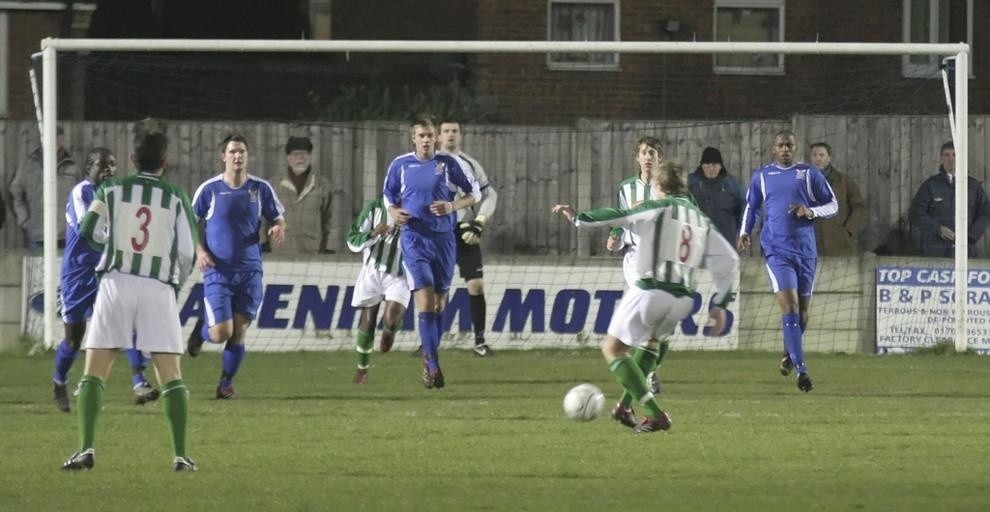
[
  {"x1": 906, "y1": 140, "x2": 989, "y2": 259},
  {"x1": 383, "y1": 118, "x2": 482, "y2": 389},
  {"x1": 186, "y1": 133, "x2": 286, "y2": 399},
  {"x1": 550, "y1": 161, "x2": 740, "y2": 434},
  {"x1": 413, "y1": 117, "x2": 498, "y2": 357},
  {"x1": 805, "y1": 143, "x2": 865, "y2": 257},
  {"x1": 606, "y1": 136, "x2": 699, "y2": 393},
  {"x1": 258, "y1": 135, "x2": 343, "y2": 256},
  {"x1": 737, "y1": 128, "x2": 840, "y2": 395},
  {"x1": 346, "y1": 198, "x2": 411, "y2": 384},
  {"x1": 686, "y1": 145, "x2": 747, "y2": 257},
  {"x1": 48, "y1": 146, "x2": 161, "y2": 413},
  {"x1": 8, "y1": 125, "x2": 82, "y2": 316},
  {"x1": 59, "y1": 126, "x2": 199, "y2": 473}
]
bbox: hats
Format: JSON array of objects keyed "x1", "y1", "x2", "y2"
[
  {"x1": 701, "y1": 147, "x2": 723, "y2": 164},
  {"x1": 286, "y1": 136, "x2": 312, "y2": 155}
]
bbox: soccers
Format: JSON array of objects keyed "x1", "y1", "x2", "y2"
[{"x1": 564, "y1": 382, "x2": 604, "y2": 421}]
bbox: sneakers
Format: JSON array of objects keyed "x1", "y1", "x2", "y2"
[
  {"x1": 353, "y1": 330, "x2": 494, "y2": 389},
  {"x1": 647, "y1": 372, "x2": 662, "y2": 394},
  {"x1": 633, "y1": 411, "x2": 672, "y2": 434},
  {"x1": 779, "y1": 352, "x2": 794, "y2": 376},
  {"x1": 612, "y1": 401, "x2": 637, "y2": 427},
  {"x1": 798, "y1": 371, "x2": 813, "y2": 391},
  {"x1": 52, "y1": 318, "x2": 239, "y2": 473}
]
